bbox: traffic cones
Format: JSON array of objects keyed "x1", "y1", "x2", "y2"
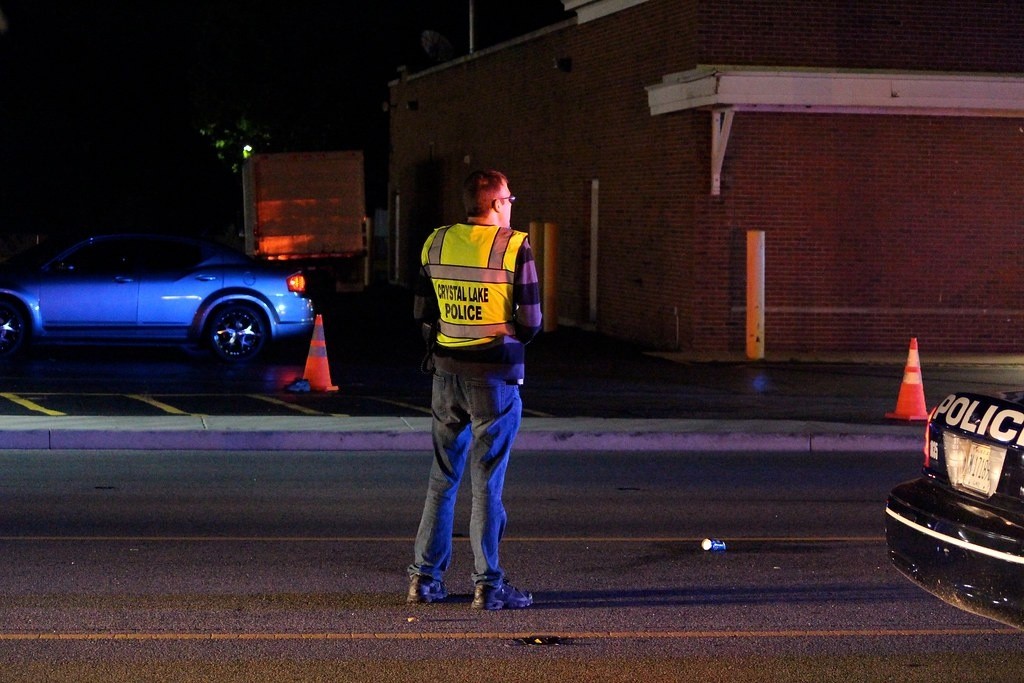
[
  {"x1": 885, "y1": 337, "x2": 931, "y2": 421},
  {"x1": 300, "y1": 313, "x2": 338, "y2": 392}
]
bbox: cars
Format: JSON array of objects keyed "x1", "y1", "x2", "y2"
[
  {"x1": 885, "y1": 390, "x2": 1024, "y2": 632},
  {"x1": 0, "y1": 232, "x2": 316, "y2": 366}
]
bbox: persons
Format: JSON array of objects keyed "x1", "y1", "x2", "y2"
[{"x1": 407, "y1": 169, "x2": 542, "y2": 609}]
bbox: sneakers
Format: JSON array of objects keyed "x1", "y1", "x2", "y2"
[
  {"x1": 471, "y1": 577, "x2": 536, "y2": 609},
  {"x1": 408, "y1": 574, "x2": 449, "y2": 605}
]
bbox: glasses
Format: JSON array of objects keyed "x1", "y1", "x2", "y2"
[{"x1": 492, "y1": 196, "x2": 517, "y2": 209}]
